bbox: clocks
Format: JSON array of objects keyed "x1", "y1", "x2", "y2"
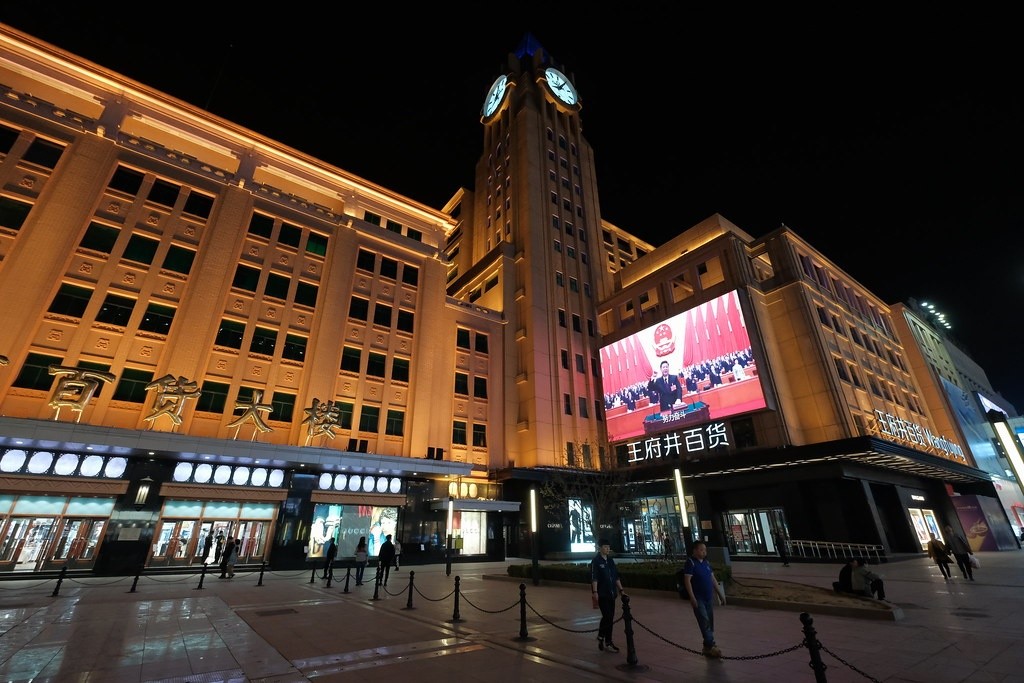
[
  {"x1": 479, "y1": 75, "x2": 507, "y2": 117},
  {"x1": 545, "y1": 67, "x2": 577, "y2": 105}
]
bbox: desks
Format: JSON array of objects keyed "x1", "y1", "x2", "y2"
[{"x1": 606, "y1": 366, "x2": 764, "y2": 438}]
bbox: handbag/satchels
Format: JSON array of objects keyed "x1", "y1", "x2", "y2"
[{"x1": 970, "y1": 555, "x2": 981, "y2": 569}]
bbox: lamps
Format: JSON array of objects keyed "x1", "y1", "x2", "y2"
[{"x1": 133, "y1": 476, "x2": 153, "y2": 512}]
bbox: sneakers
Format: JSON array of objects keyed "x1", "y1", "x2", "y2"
[
  {"x1": 605, "y1": 643, "x2": 619, "y2": 652},
  {"x1": 596, "y1": 635, "x2": 605, "y2": 652}
]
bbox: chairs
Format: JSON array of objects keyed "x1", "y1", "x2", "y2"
[
  {"x1": 728, "y1": 377, "x2": 734, "y2": 382},
  {"x1": 752, "y1": 370, "x2": 758, "y2": 376},
  {"x1": 703, "y1": 385, "x2": 711, "y2": 391}
]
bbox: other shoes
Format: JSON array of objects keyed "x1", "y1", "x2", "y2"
[
  {"x1": 949, "y1": 575, "x2": 951, "y2": 577},
  {"x1": 227, "y1": 574, "x2": 235, "y2": 579},
  {"x1": 218, "y1": 575, "x2": 226, "y2": 579},
  {"x1": 944, "y1": 575, "x2": 947, "y2": 579},
  {"x1": 360, "y1": 581, "x2": 363, "y2": 585},
  {"x1": 879, "y1": 599, "x2": 891, "y2": 604},
  {"x1": 327, "y1": 577, "x2": 332, "y2": 579},
  {"x1": 379, "y1": 583, "x2": 382, "y2": 585},
  {"x1": 963, "y1": 572, "x2": 967, "y2": 579},
  {"x1": 384, "y1": 583, "x2": 386, "y2": 586},
  {"x1": 969, "y1": 576, "x2": 974, "y2": 581},
  {"x1": 321, "y1": 575, "x2": 326, "y2": 579},
  {"x1": 356, "y1": 582, "x2": 359, "y2": 586},
  {"x1": 702, "y1": 645, "x2": 721, "y2": 657}
]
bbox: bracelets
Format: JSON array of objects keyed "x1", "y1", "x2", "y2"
[
  {"x1": 620, "y1": 589, "x2": 623, "y2": 592},
  {"x1": 593, "y1": 591, "x2": 598, "y2": 593}
]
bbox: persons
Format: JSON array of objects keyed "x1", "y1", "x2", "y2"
[
  {"x1": 394, "y1": 540, "x2": 401, "y2": 570},
  {"x1": 638, "y1": 533, "x2": 644, "y2": 554},
  {"x1": 197, "y1": 531, "x2": 241, "y2": 578},
  {"x1": 355, "y1": 537, "x2": 368, "y2": 586},
  {"x1": 928, "y1": 526, "x2": 974, "y2": 581},
  {"x1": 570, "y1": 502, "x2": 581, "y2": 543},
  {"x1": 664, "y1": 534, "x2": 673, "y2": 561},
  {"x1": 379, "y1": 535, "x2": 395, "y2": 585},
  {"x1": 685, "y1": 541, "x2": 721, "y2": 655},
  {"x1": 839, "y1": 557, "x2": 891, "y2": 603},
  {"x1": 604, "y1": 345, "x2": 755, "y2": 412},
  {"x1": 775, "y1": 533, "x2": 789, "y2": 567},
  {"x1": 1021, "y1": 527, "x2": 1024, "y2": 541},
  {"x1": 322, "y1": 538, "x2": 336, "y2": 579},
  {"x1": 591, "y1": 539, "x2": 625, "y2": 652}
]
